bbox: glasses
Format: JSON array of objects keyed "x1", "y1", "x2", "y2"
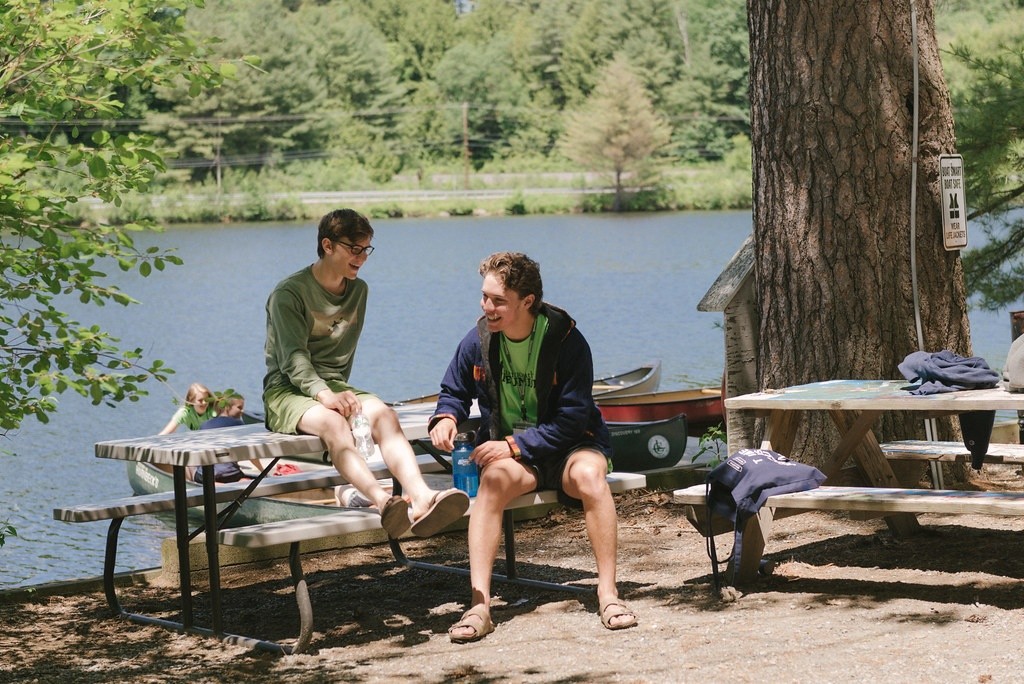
[{"x1": 334, "y1": 240, "x2": 375, "y2": 256}]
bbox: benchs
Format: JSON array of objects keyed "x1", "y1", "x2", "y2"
[
  {"x1": 673, "y1": 439, "x2": 1024, "y2": 602},
  {"x1": 53, "y1": 451, "x2": 647, "y2": 653}
]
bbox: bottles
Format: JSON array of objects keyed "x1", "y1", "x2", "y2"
[{"x1": 451, "y1": 429, "x2": 479, "y2": 497}]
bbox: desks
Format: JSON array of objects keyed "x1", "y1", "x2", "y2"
[
  {"x1": 95, "y1": 399, "x2": 521, "y2": 628},
  {"x1": 724, "y1": 380, "x2": 1024, "y2": 585}
]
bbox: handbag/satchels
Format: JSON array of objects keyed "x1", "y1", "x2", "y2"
[
  {"x1": 273, "y1": 464, "x2": 304, "y2": 476},
  {"x1": 706, "y1": 448, "x2": 828, "y2": 598}
]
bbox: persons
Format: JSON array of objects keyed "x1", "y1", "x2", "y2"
[
  {"x1": 157, "y1": 383, "x2": 245, "y2": 435},
  {"x1": 429, "y1": 251, "x2": 637, "y2": 641},
  {"x1": 184, "y1": 393, "x2": 267, "y2": 484},
  {"x1": 263, "y1": 208, "x2": 471, "y2": 539}
]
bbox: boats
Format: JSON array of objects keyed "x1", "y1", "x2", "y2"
[
  {"x1": 242, "y1": 410, "x2": 687, "y2": 469},
  {"x1": 591, "y1": 386, "x2": 726, "y2": 430},
  {"x1": 386, "y1": 358, "x2": 663, "y2": 408},
  {"x1": 126, "y1": 456, "x2": 393, "y2": 528}
]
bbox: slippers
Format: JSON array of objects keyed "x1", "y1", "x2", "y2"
[
  {"x1": 448, "y1": 609, "x2": 494, "y2": 643},
  {"x1": 381, "y1": 495, "x2": 411, "y2": 539},
  {"x1": 411, "y1": 489, "x2": 470, "y2": 538},
  {"x1": 599, "y1": 600, "x2": 638, "y2": 629}
]
